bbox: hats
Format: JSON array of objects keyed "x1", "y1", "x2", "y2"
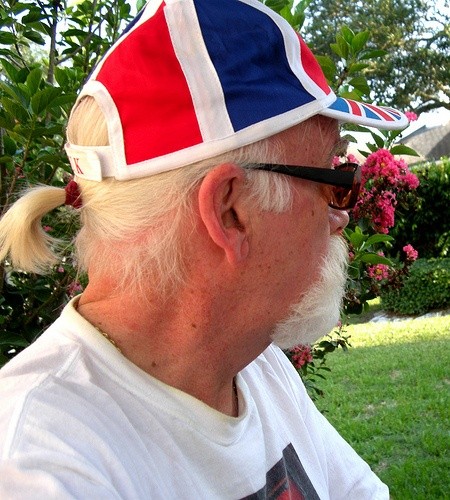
[{"x1": 63, "y1": 1, "x2": 410, "y2": 183}]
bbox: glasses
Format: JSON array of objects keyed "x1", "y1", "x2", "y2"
[{"x1": 237, "y1": 161, "x2": 361, "y2": 210}]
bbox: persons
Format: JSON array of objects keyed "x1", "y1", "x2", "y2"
[{"x1": 0, "y1": 0, "x2": 418, "y2": 500}]
